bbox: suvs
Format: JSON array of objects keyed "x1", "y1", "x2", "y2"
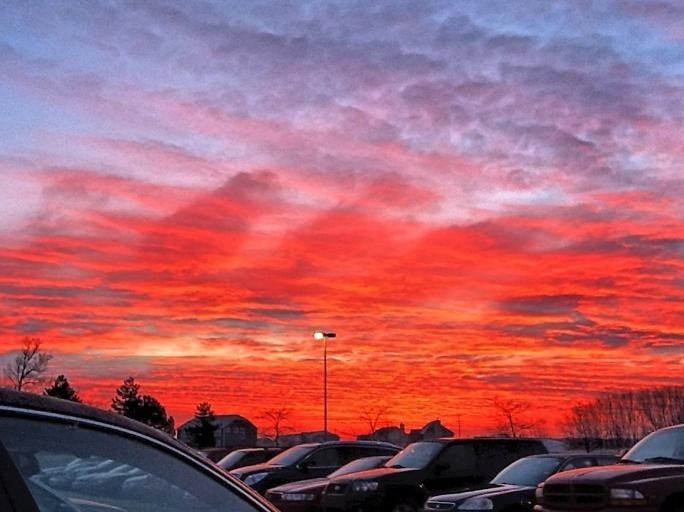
[
  {"x1": 533, "y1": 423, "x2": 684, "y2": 512},
  {"x1": 319, "y1": 436, "x2": 549, "y2": 512}
]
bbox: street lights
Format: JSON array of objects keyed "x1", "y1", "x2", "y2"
[{"x1": 313, "y1": 330, "x2": 336, "y2": 441}]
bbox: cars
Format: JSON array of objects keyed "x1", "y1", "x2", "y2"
[
  {"x1": 0, "y1": 394, "x2": 281, "y2": 512},
  {"x1": 265, "y1": 455, "x2": 394, "y2": 510},
  {"x1": 212, "y1": 448, "x2": 282, "y2": 473},
  {"x1": 228, "y1": 439, "x2": 405, "y2": 496},
  {"x1": 26, "y1": 453, "x2": 198, "y2": 502},
  {"x1": 424, "y1": 450, "x2": 624, "y2": 511}
]
